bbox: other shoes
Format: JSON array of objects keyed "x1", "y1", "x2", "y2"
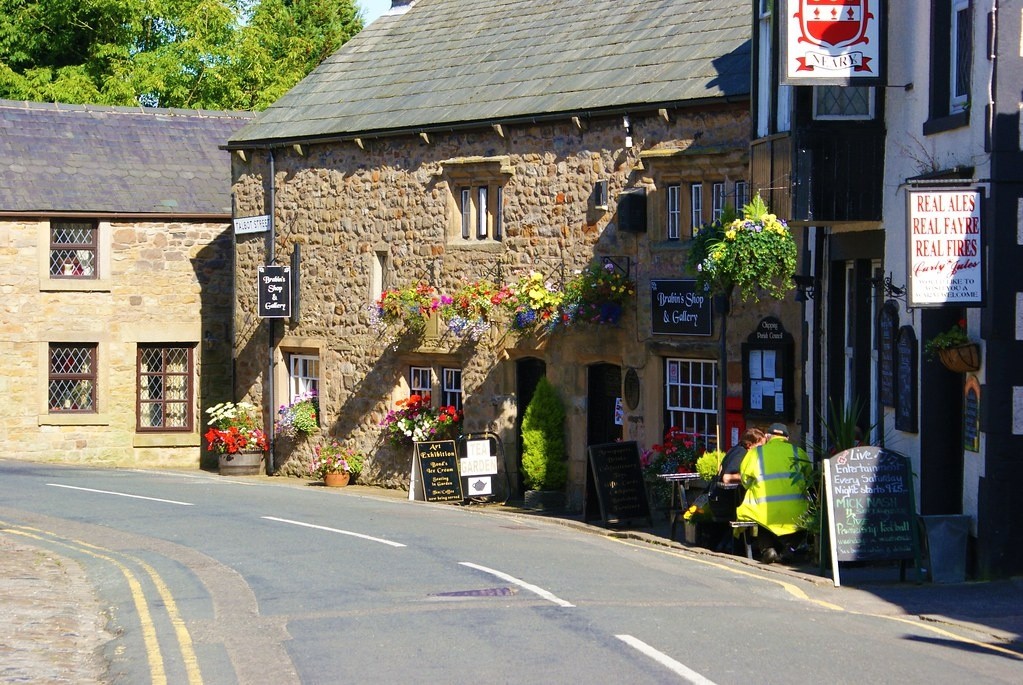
[
  {"x1": 781, "y1": 547, "x2": 808, "y2": 563},
  {"x1": 761, "y1": 548, "x2": 781, "y2": 564}
]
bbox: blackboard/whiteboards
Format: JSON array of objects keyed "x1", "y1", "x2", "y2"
[
  {"x1": 584, "y1": 441, "x2": 648, "y2": 522},
  {"x1": 965, "y1": 375, "x2": 980, "y2": 453},
  {"x1": 876, "y1": 304, "x2": 899, "y2": 407},
  {"x1": 819, "y1": 445, "x2": 921, "y2": 562},
  {"x1": 893, "y1": 325, "x2": 918, "y2": 433}
]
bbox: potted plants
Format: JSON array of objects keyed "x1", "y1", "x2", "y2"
[
  {"x1": 519, "y1": 372, "x2": 570, "y2": 511},
  {"x1": 881, "y1": 129, "x2": 976, "y2": 188}
]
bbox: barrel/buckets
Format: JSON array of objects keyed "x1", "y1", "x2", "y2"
[{"x1": 919, "y1": 514, "x2": 972, "y2": 584}]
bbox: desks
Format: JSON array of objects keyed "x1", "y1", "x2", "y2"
[
  {"x1": 717, "y1": 481, "x2": 743, "y2": 490},
  {"x1": 655, "y1": 473, "x2": 701, "y2": 540}
]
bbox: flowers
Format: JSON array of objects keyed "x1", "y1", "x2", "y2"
[
  {"x1": 924, "y1": 318, "x2": 967, "y2": 365},
  {"x1": 639, "y1": 426, "x2": 706, "y2": 508},
  {"x1": 204, "y1": 402, "x2": 270, "y2": 455},
  {"x1": 366, "y1": 280, "x2": 441, "y2": 353},
  {"x1": 273, "y1": 389, "x2": 319, "y2": 443},
  {"x1": 432, "y1": 277, "x2": 514, "y2": 342},
  {"x1": 499, "y1": 271, "x2": 565, "y2": 333},
  {"x1": 379, "y1": 395, "x2": 464, "y2": 451},
  {"x1": 307, "y1": 437, "x2": 366, "y2": 481},
  {"x1": 542, "y1": 261, "x2": 636, "y2": 333},
  {"x1": 683, "y1": 191, "x2": 798, "y2": 305}
]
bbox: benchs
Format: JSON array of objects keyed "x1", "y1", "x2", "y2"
[{"x1": 729, "y1": 520, "x2": 758, "y2": 561}]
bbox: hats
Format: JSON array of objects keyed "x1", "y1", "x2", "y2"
[{"x1": 767, "y1": 423, "x2": 789, "y2": 437}]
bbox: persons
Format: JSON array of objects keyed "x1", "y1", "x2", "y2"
[
  {"x1": 709, "y1": 429, "x2": 766, "y2": 556},
  {"x1": 733, "y1": 423, "x2": 820, "y2": 565}
]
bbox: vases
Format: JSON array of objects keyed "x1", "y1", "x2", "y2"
[
  {"x1": 648, "y1": 508, "x2": 670, "y2": 530},
  {"x1": 217, "y1": 449, "x2": 262, "y2": 476},
  {"x1": 323, "y1": 470, "x2": 351, "y2": 487},
  {"x1": 920, "y1": 514, "x2": 973, "y2": 585},
  {"x1": 938, "y1": 342, "x2": 980, "y2": 373}
]
